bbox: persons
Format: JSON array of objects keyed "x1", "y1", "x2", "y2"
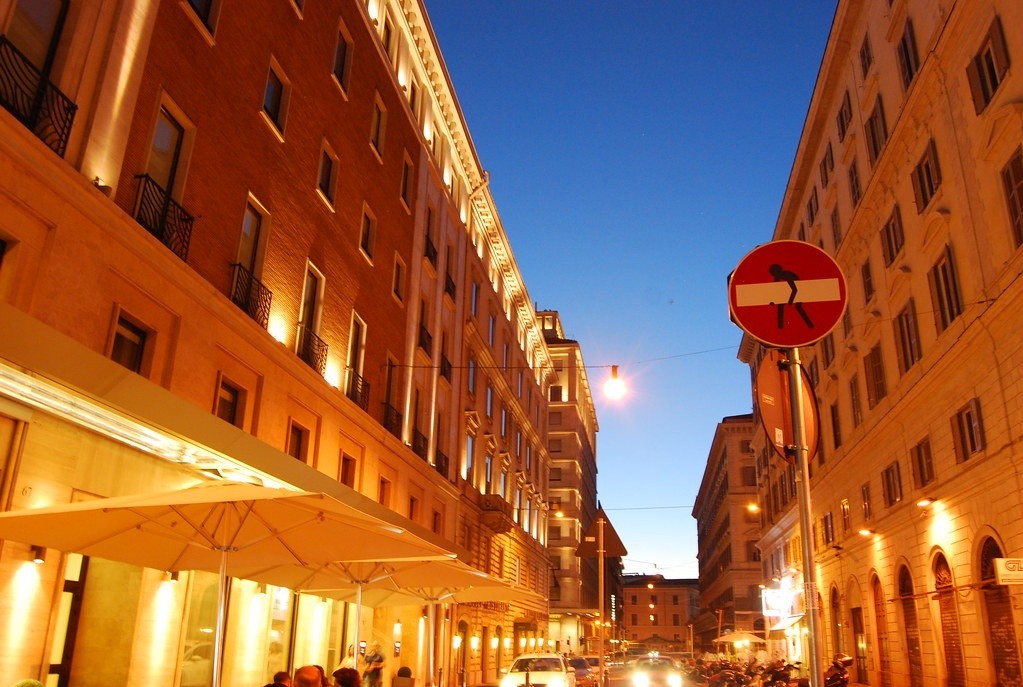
[
  {"x1": 341, "y1": 643, "x2": 362, "y2": 670},
  {"x1": 824, "y1": 653, "x2": 850, "y2": 687},
  {"x1": 263, "y1": 665, "x2": 360, "y2": 687},
  {"x1": 363, "y1": 640, "x2": 387, "y2": 687},
  {"x1": 681, "y1": 657, "x2": 793, "y2": 687}
]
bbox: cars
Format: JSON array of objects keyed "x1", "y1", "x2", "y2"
[
  {"x1": 499, "y1": 651, "x2": 577, "y2": 687},
  {"x1": 628, "y1": 655, "x2": 684, "y2": 687},
  {"x1": 568, "y1": 655, "x2": 601, "y2": 687},
  {"x1": 583, "y1": 650, "x2": 642, "y2": 687}
]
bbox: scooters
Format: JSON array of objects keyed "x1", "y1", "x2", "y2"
[{"x1": 678, "y1": 651, "x2": 854, "y2": 687}]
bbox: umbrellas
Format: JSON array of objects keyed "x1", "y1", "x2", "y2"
[
  {"x1": 714, "y1": 632, "x2": 765, "y2": 643},
  {"x1": 298, "y1": 558, "x2": 544, "y2": 687},
  {"x1": 0, "y1": 477, "x2": 457, "y2": 687}
]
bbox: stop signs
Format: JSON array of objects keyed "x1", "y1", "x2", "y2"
[{"x1": 730, "y1": 237, "x2": 849, "y2": 348}]
